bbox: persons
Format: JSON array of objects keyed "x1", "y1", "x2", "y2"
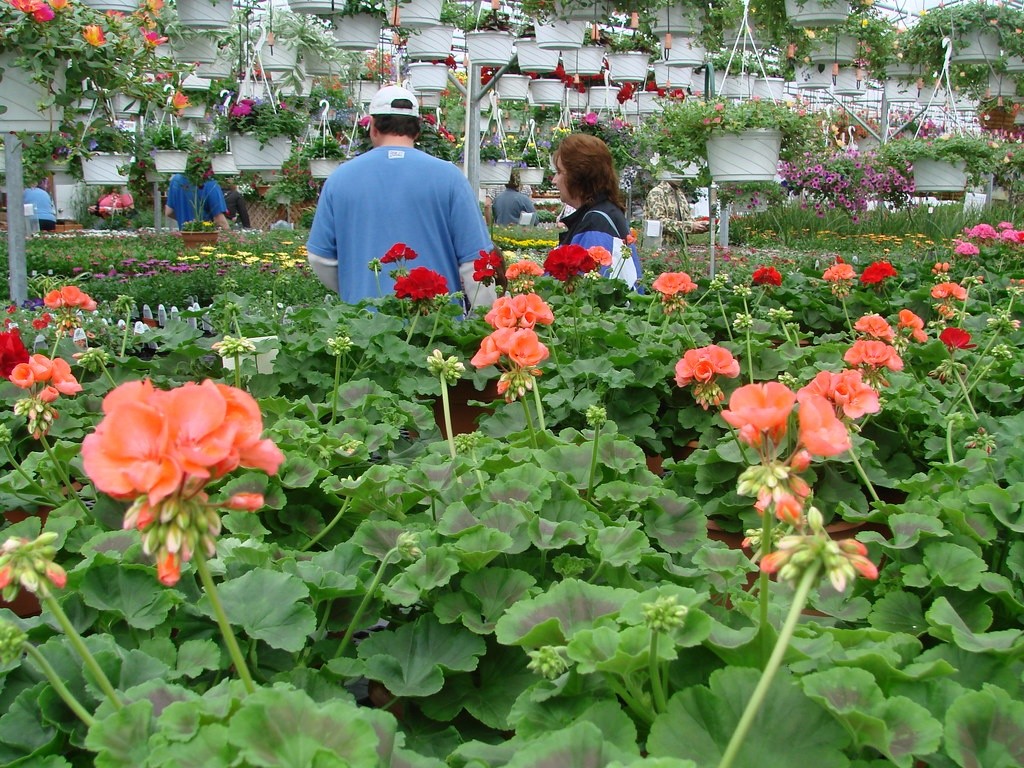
[
  {"x1": 492, "y1": 171, "x2": 539, "y2": 226},
  {"x1": 307, "y1": 86, "x2": 497, "y2": 323},
  {"x1": 88, "y1": 185, "x2": 134, "y2": 231},
  {"x1": 643, "y1": 180, "x2": 710, "y2": 245},
  {"x1": 556, "y1": 204, "x2": 577, "y2": 228},
  {"x1": 165, "y1": 151, "x2": 230, "y2": 233},
  {"x1": 542, "y1": 134, "x2": 645, "y2": 306},
  {"x1": 459, "y1": 241, "x2": 510, "y2": 317},
  {"x1": 220, "y1": 179, "x2": 251, "y2": 229},
  {"x1": 22, "y1": 176, "x2": 56, "y2": 232},
  {"x1": 485, "y1": 184, "x2": 534, "y2": 227}
]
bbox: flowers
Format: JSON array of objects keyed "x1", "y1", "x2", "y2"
[
  {"x1": 0, "y1": 0, "x2": 1024, "y2": 222},
  {"x1": 0, "y1": 223, "x2": 1023, "y2": 768}
]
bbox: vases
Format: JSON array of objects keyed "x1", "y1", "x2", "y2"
[
  {"x1": 77, "y1": 0, "x2": 1024, "y2": 249},
  {"x1": 408, "y1": 380, "x2": 501, "y2": 440},
  {"x1": 0, "y1": 482, "x2": 83, "y2": 619},
  {"x1": 706, "y1": 518, "x2": 760, "y2": 608},
  {"x1": 802, "y1": 521, "x2": 867, "y2": 616}
]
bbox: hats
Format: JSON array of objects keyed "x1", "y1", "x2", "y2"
[{"x1": 369, "y1": 84, "x2": 420, "y2": 119}]
